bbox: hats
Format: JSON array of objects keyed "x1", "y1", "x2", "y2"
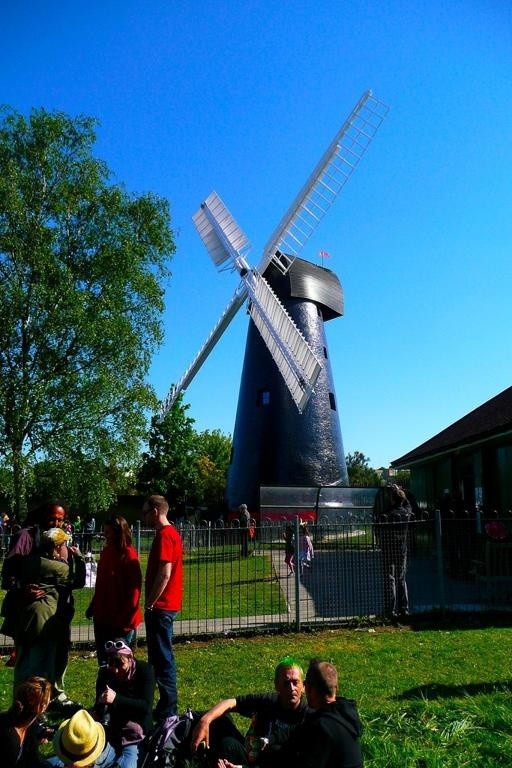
[
  {"x1": 106, "y1": 646, "x2": 133, "y2": 655},
  {"x1": 53, "y1": 708, "x2": 106, "y2": 767},
  {"x1": 40, "y1": 527, "x2": 72, "y2": 549}
]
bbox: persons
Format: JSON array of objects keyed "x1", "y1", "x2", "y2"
[
  {"x1": 373, "y1": 482, "x2": 412, "y2": 619},
  {"x1": 0, "y1": 641, "x2": 364, "y2": 768},
  {"x1": 1, "y1": 492, "x2": 184, "y2": 711},
  {"x1": 215, "y1": 503, "x2": 250, "y2": 556},
  {"x1": 280, "y1": 522, "x2": 316, "y2": 578}
]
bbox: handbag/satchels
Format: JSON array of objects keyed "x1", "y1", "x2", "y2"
[{"x1": 143, "y1": 710, "x2": 194, "y2": 767}]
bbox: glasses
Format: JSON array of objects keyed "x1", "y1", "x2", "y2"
[{"x1": 104, "y1": 641, "x2": 132, "y2": 652}]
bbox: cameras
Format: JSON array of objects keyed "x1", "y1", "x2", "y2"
[
  {"x1": 259, "y1": 737, "x2": 269, "y2": 751},
  {"x1": 34, "y1": 721, "x2": 59, "y2": 740}
]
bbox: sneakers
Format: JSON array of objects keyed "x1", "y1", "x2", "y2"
[
  {"x1": 39, "y1": 725, "x2": 54, "y2": 744},
  {"x1": 150, "y1": 712, "x2": 171, "y2": 722},
  {"x1": 51, "y1": 702, "x2": 82, "y2": 718}
]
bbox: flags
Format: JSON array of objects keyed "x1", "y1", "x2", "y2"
[{"x1": 320, "y1": 249, "x2": 330, "y2": 256}]
